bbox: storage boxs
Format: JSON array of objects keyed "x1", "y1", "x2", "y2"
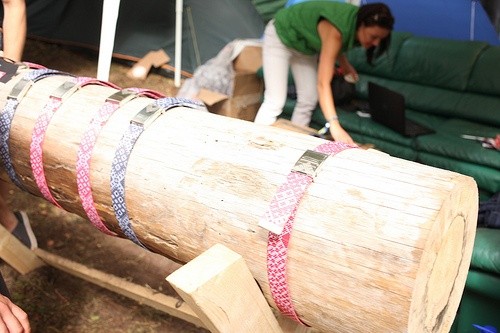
[{"x1": 196, "y1": 47, "x2": 262, "y2": 123}]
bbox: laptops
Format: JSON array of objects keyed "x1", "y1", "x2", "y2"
[{"x1": 368, "y1": 80, "x2": 437, "y2": 137}]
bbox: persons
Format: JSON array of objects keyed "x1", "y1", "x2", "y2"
[
  {"x1": 0, "y1": 0, "x2": 69, "y2": 265},
  {"x1": 0, "y1": 295, "x2": 31, "y2": 333},
  {"x1": 253, "y1": 0, "x2": 395, "y2": 147}
]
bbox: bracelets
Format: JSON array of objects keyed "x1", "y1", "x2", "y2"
[
  {"x1": 4, "y1": 57, "x2": 15, "y2": 62},
  {"x1": 326, "y1": 115, "x2": 338, "y2": 123}
]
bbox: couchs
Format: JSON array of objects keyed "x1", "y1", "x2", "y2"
[{"x1": 257, "y1": 33, "x2": 500, "y2": 333}]
